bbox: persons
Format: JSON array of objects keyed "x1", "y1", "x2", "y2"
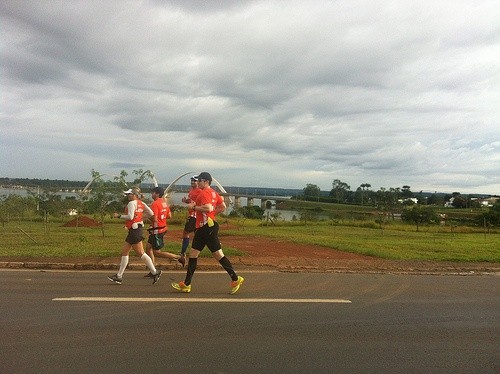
[
  {"x1": 178, "y1": 176, "x2": 199, "y2": 254},
  {"x1": 144, "y1": 187, "x2": 185, "y2": 279},
  {"x1": 105, "y1": 187, "x2": 163, "y2": 285},
  {"x1": 172, "y1": 171, "x2": 244, "y2": 295}
]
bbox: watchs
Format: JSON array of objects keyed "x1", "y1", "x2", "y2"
[{"x1": 193, "y1": 205, "x2": 196, "y2": 210}]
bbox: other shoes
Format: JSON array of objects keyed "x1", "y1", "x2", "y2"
[
  {"x1": 107, "y1": 273, "x2": 122, "y2": 285},
  {"x1": 152, "y1": 270, "x2": 163, "y2": 285},
  {"x1": 178, "y1": 256, "x2": 185, "y2": 267},
  {"x1": 171, "y1": 281, "x2": 192, "y2": 292},
  {"x1": 144, "y1": 272, "x2": 156, "y2": 278},
  {"x1": 231, "y1": 275, "x2": 244, "y2": 294}
]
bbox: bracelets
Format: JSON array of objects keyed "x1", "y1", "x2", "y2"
[{"x1": 117, "y1": 215, "x2": 121, "y2": 218}]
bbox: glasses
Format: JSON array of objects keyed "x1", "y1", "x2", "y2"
[{"x1": 198, "y1": 179, "x2": 206, "y2": 182}]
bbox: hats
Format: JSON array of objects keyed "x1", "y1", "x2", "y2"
[
  {"x1": 190, "y1": 175, "x2": 199, "y2": 181},
  {"x1": 193, "y1": 172, "x2": 211, "y2": 181},
  {"x1": 124, "y1": 187, "x2": 141, "y2": 195}
]
